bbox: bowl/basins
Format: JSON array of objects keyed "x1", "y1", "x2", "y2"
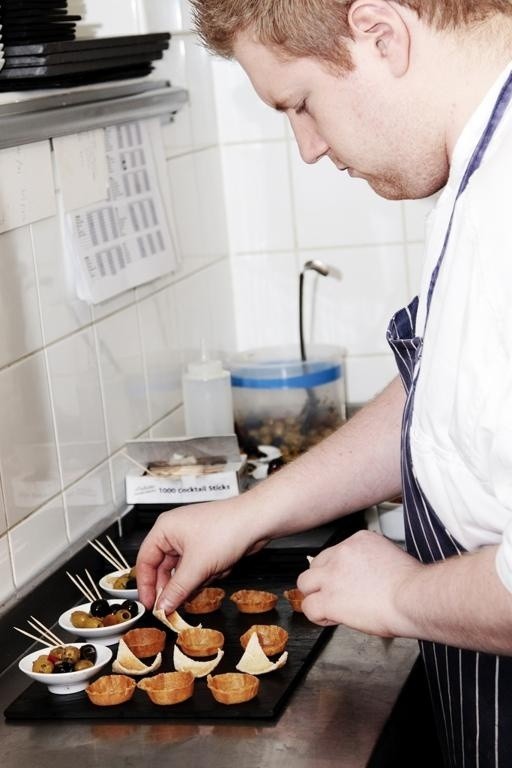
[
  {"x1": 98, "y1": 566, "x2": 177, "y2": 599},
  {"x1": 58, "y1": 598, "x2": 147, "y2": 647},
  {"x1": 19, "y1": 642, "x2": 114, "y2": 696}
]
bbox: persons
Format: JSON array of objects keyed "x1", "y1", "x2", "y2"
[{"x1": 132, "y1": 0, "x2": 512, "y2": 768}]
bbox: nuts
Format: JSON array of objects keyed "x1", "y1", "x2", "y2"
[{"x1": 249, "y1": 420, "x2": 284, "y2": 444}]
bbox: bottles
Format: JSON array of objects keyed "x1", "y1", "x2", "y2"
[{"x1": 183, "y1": 339, "x2": 236, "y2": 440}]
